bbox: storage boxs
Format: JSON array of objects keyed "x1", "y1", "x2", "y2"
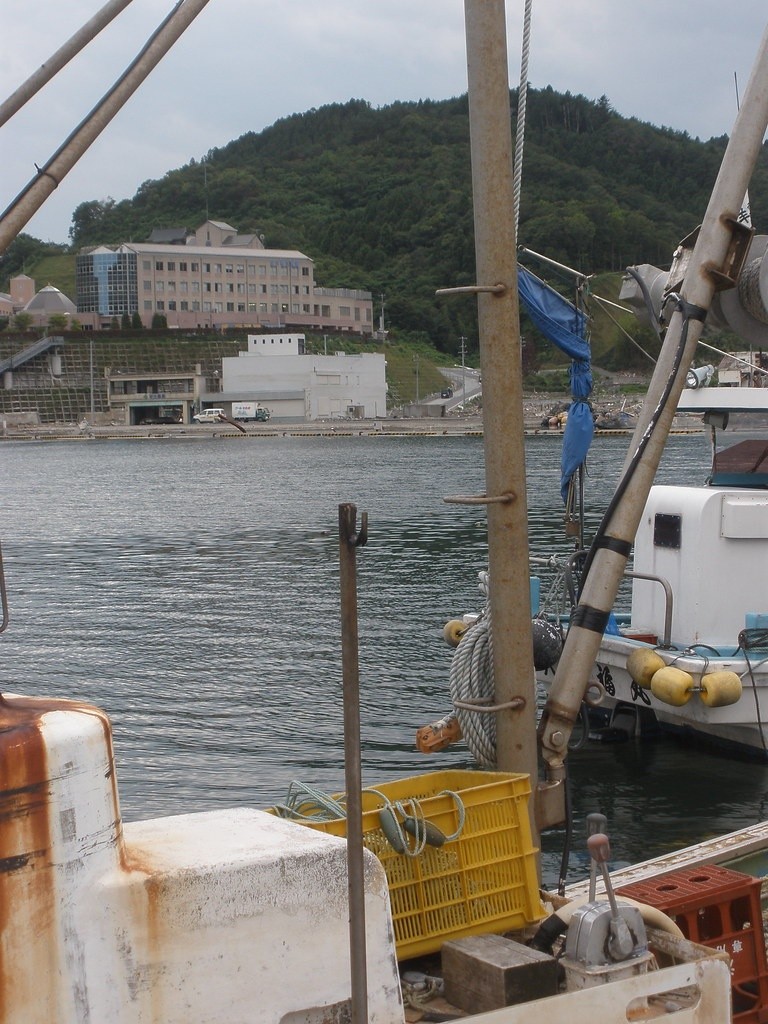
[
  {"x1": 619, "y1": 863, "x2": 768, "y2": 1024},
  {"x1": 439, "y1": 932, "x2": 558, "y2": 1014},
  {"x1": 265, "y1": 769, "x2": 549, "y2": 957}
]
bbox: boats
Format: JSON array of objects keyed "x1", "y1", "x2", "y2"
[{"x1": 540, "y1": 343, "x2": 768, "y2": 756}]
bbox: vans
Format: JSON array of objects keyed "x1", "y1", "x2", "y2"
[{"x1": 194, "y1": 409, "x2": 226, "y2": 425}]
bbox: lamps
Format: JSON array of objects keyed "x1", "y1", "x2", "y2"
[{"x1": 685, "y1": 364, "x2": 715, "y2": 389}]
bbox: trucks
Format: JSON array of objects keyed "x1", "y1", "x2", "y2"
[{"x1": 232, "y1": 402, "x2": 273, "y2": 423}]
